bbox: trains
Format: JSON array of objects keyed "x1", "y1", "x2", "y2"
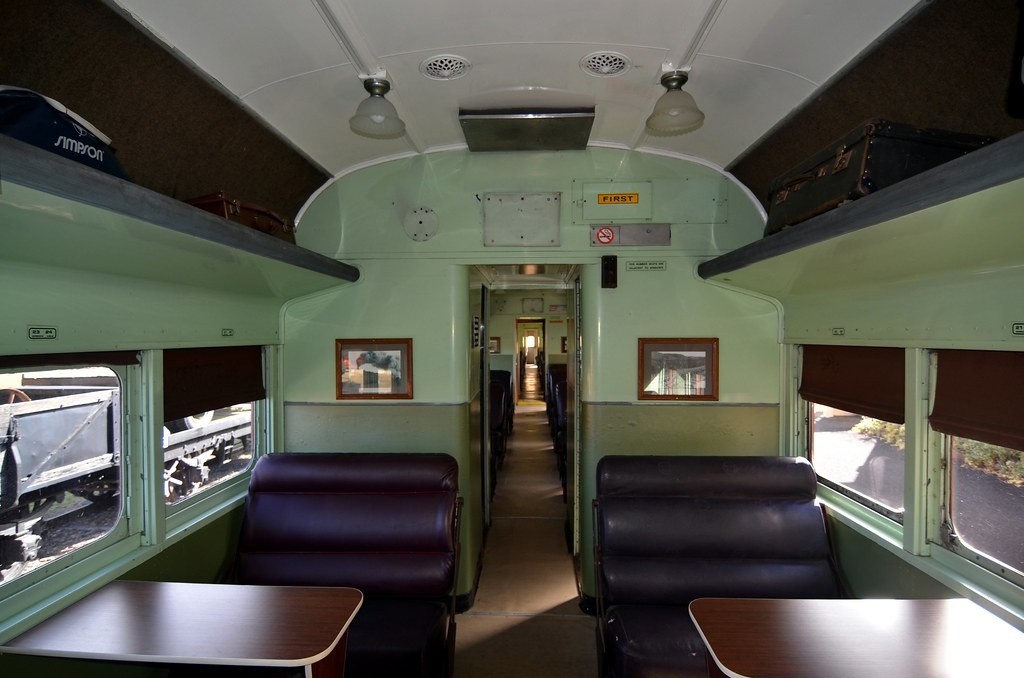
[{"x1": 0, "y1": 366, "x2": 253, "y2": 564}]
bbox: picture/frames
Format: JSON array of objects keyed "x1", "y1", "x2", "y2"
[
  {"x1": 490, "y1": 337, "x2": 500, "y2": 353},
  {"x1": 638, "y1": 338, "x2": 719, "y2": 402},
  {"x1": 561, "y1": 336, "x2": 568, "y2": 352},
  {"x1": 334, "y1": 339, "x2": 413, "y2": 399}
]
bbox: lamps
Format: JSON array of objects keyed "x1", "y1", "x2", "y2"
[
  {"x1": 349, "y1": 79, "x2": 407, "y2": 137},
  {"x1": 646, "y1": 69, "x2": 705, "y2": 137}
]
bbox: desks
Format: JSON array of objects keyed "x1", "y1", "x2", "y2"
[
  {"x1": 0, "y1": 579, "x2": 364, "y2": 678},
  {"x1": 689, "y1": 596, "x2": 1024, "y2": 678}
]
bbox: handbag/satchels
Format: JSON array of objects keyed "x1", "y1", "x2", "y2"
[{"x1": 0, "y1": 84, "x2": 131, "y2": 181}]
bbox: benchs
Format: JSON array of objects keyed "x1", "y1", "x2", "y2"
[
  {"x1": 536, "y1": 352, "x2": 568, "y2": 490},
  {"x1": 224, "y1": 453, "x2": 462, "y2": 677},
  {"x1": 592, "y1": 454, "x2": 857, "y2": 677},
  {"x1": 489, "y1": 369, "x2": 514, "y2": 483}
]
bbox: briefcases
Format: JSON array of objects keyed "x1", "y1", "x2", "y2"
[{"x1": 185, "y1": 189, "x2": 296, "y2": 246}]
